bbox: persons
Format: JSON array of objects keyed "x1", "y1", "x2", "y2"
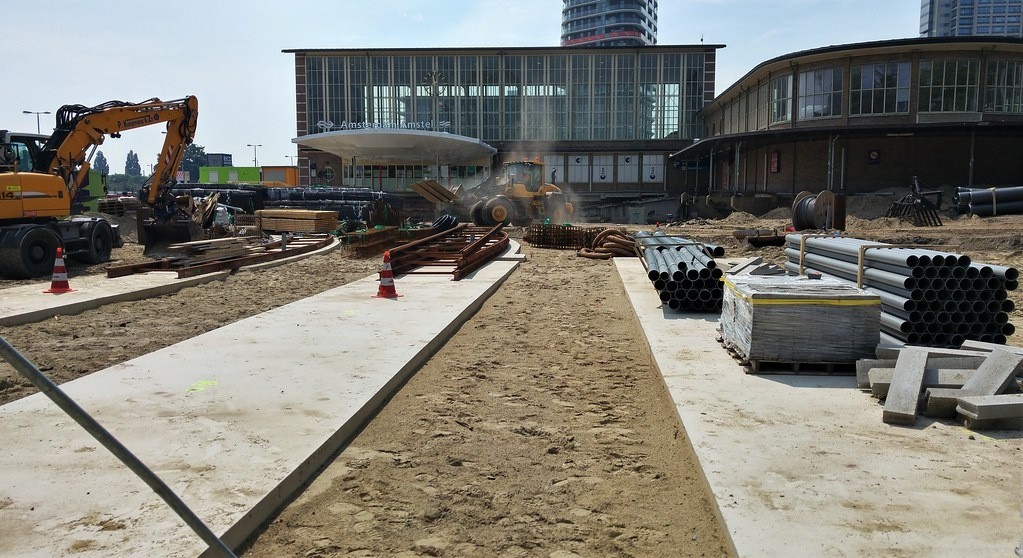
[
  {"x1": 20, "y1": 150, "x2": 31, "y2": 171},
  {"x1": 518, "y1": 168, "x2": 530, "y2": 184}
]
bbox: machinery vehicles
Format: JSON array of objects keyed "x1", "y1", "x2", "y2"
[
  {"x1": 0, "y1": 95, "x2": 205, "y2": 280},
  {"x1": 470, "y1": 157, "x2": 574, "y2": 227}
]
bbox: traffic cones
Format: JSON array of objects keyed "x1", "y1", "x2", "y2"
[
  {"x1": 42, "y1": 248, "x2": 79, "y2": 293},
  {"x1": 371, "y1": 251, "x2": 404, "y2": 297}
]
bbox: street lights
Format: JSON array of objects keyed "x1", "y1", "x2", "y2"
[
  {"x1": 247, "y1": 144, "x2": 262, "y2": 167},
  {"x1": 22, "y1": 111, "x2": 51, "y2": 149},
  {"x1": 285, "y1": 155, "x2": 298, "y2": 167}
]
